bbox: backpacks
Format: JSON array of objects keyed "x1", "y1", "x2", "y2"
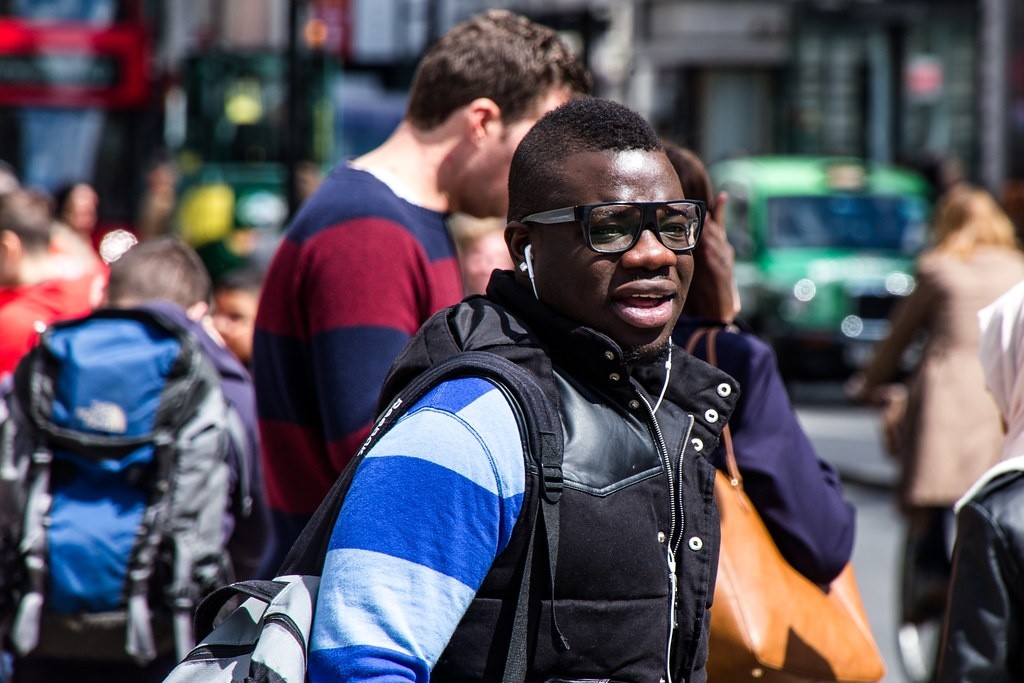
[
  {"x1": 0, "y1": 308, "x2": 238, "y2": 667},
  {"x1": 162, "y1": 352, "x2": 564, "y2": 683}
]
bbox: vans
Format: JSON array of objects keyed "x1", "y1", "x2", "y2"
[{"x1": 707, "y1": 155, "x2": 934, "y2": 401}]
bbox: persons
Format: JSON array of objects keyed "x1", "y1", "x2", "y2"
[
  {"x1": 848, "y1": 140, "x2": 1024, "y2": 683},
  {"x1": 163, "y1": 98, "x2": 743, "y2": 682},
  {"x1": 248, "y1": 10, "x2": 597, "y2": 574},
  {"x1": 614, "y1": 138, "x2": 888, "y2": 683},
  {"x1": 448, "y1": 210, "x2": 520, "y2": 298},
  {"x1": 0, "y1": 158, "x2": 279, "y2": 683}
]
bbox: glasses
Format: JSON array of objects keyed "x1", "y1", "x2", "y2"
[{"x1": 519, "y1": 201, "x2": 705, "y2": 255}]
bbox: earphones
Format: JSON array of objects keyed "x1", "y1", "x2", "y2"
[{"x1": 525, "y1": 244, "x2": 534, "y2": 280}]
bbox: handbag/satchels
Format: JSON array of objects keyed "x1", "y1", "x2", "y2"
[{"x1": 680, "y1": 329, "x2": 884, "y2": 683}]
